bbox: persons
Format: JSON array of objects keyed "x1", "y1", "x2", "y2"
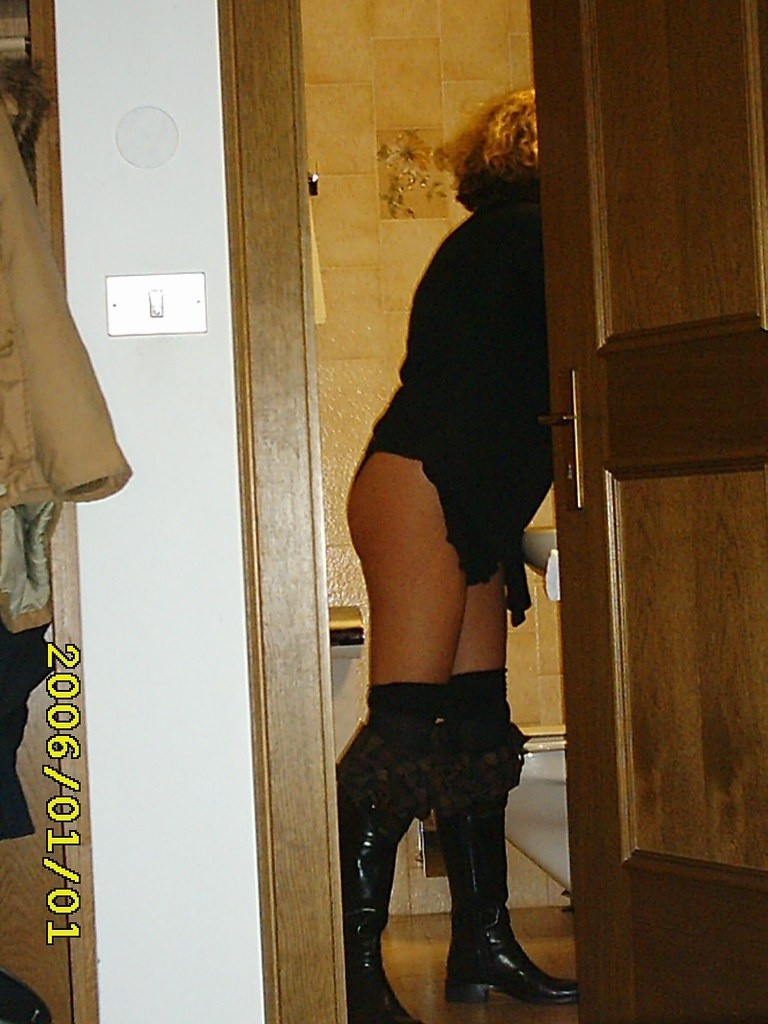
[{"x1": 334, "y1": 85, "x2": 578, "y2": 1024}]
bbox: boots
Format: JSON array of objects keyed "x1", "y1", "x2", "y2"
[
  {"x1": 340, "y1": 786, "x2": 424, "y2": 1023},
  {"x1": 443, "y1": 812, "x2": 581, "y2": 1005}
]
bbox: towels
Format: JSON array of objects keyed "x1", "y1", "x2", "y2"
[{"x1": 308, "y1": 195, "x2": 327, "y2": 330}]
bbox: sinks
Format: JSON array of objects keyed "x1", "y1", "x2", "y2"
[{"x1": 521, "y1": 527, "x2": 557, "y2": 572}]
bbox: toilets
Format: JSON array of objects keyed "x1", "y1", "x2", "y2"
[{"x1": 501, "y1": 724, "x2": 571, "y2": 890}]
